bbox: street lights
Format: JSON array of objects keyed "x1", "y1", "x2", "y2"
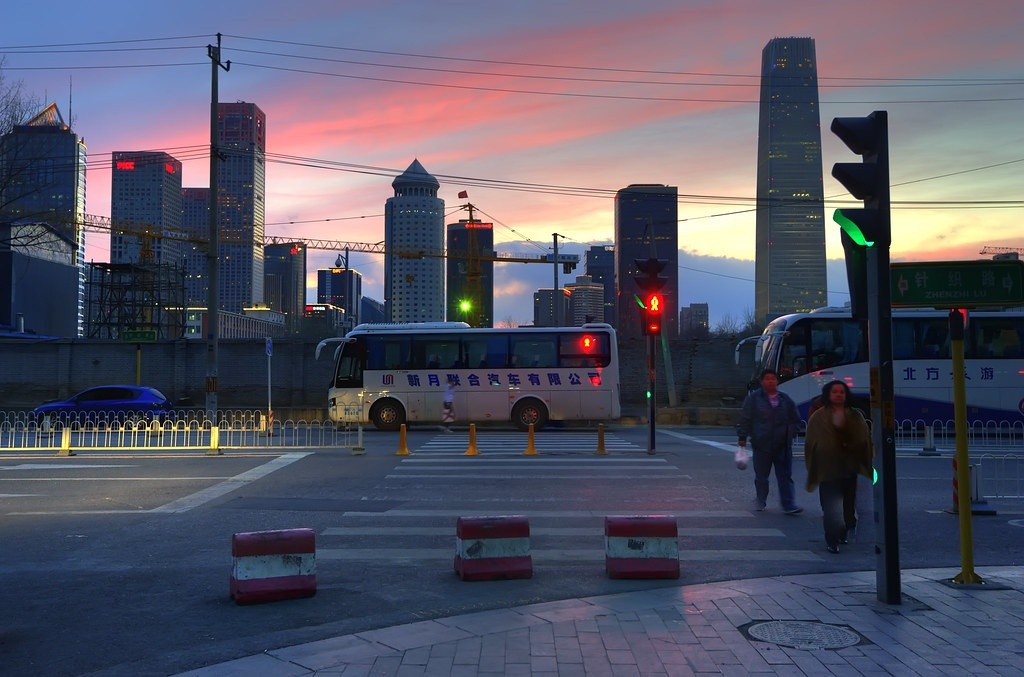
[{"x1": 460, "y1": 299, "x2": 473, "y2": 323}]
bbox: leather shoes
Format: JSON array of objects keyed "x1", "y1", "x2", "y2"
[
  {"x1": 838, "y1": 534, "x2": 849, "y2": 544},
  {"x1": 827, "y1": 543, "x2": 840, "y2": 552}
]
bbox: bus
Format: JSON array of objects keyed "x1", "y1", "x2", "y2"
[
  {"x1": 314, "y1": 320, "x2": 624, "y2": 432},
  {"x1": 734, "y1": 304, "x2": 1024, "y2": 436},
  {"x1": 304, "y1": 304, "x2": 345, "y2": 320}
]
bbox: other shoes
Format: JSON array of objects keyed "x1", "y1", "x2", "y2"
[
  {"x1": 781, "y1": 504, "x2": 803, "y2": 512},
  {"x1": 753, "y1": 502, "x2": 765, "y2": 511}
]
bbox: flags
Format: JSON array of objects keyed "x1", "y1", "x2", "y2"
[{"x1": 458, "y1": 190, "x2": 468, "y2": 198}]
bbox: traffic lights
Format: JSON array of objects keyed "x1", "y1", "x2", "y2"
[
  {"x1": 651, "y1": 258, "x2": 668, "y2": 292},
  {"x1": 833, "y1": 109, "x2": 894, "y2": 246},
  {"x1": 634, "y1": 257, "x2": 650, "y2": 291},
  {"x1": 644, "y1": 293, "x2": 663, "y2": 336}
]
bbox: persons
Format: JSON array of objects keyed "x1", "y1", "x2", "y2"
[
  {"x1": 805, "y1": 381, "x2": 872, "y2": 553},
  {"x1": 439, "y1": 378, "x2": 456, "y2": 434},
  {"x1": 797, "y1": 341, "x2": 833, "y2": 374},
  {"x1": 737, "y1": 369, "x2": 804, "y2": 515}
]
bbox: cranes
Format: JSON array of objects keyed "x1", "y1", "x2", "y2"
[
  {"x1": 264, "y1": 204, "x2": 580, "y2": 329},
  {"x1": 62, "y1": 212, "x2": 272, "y2": 337},
  {"x1": 979, "y1": 245, "x2": 1024, "y2": 257}
]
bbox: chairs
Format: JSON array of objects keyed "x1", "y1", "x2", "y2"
[
  {"x1": 839, "y1": 343, "x2": 1024, "y2": 364},
  {"x1": 404, "y1": 351, "x2": 540, "y2": 369}
]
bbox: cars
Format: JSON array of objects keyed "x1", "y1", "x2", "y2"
[{"x1": 30, "y1": 384, "x2": 177, "y2": 430}]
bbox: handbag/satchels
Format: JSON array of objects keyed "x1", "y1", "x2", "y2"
[{"x1": 735, "y1": 446, "x2": 753, "y2": 469}]
